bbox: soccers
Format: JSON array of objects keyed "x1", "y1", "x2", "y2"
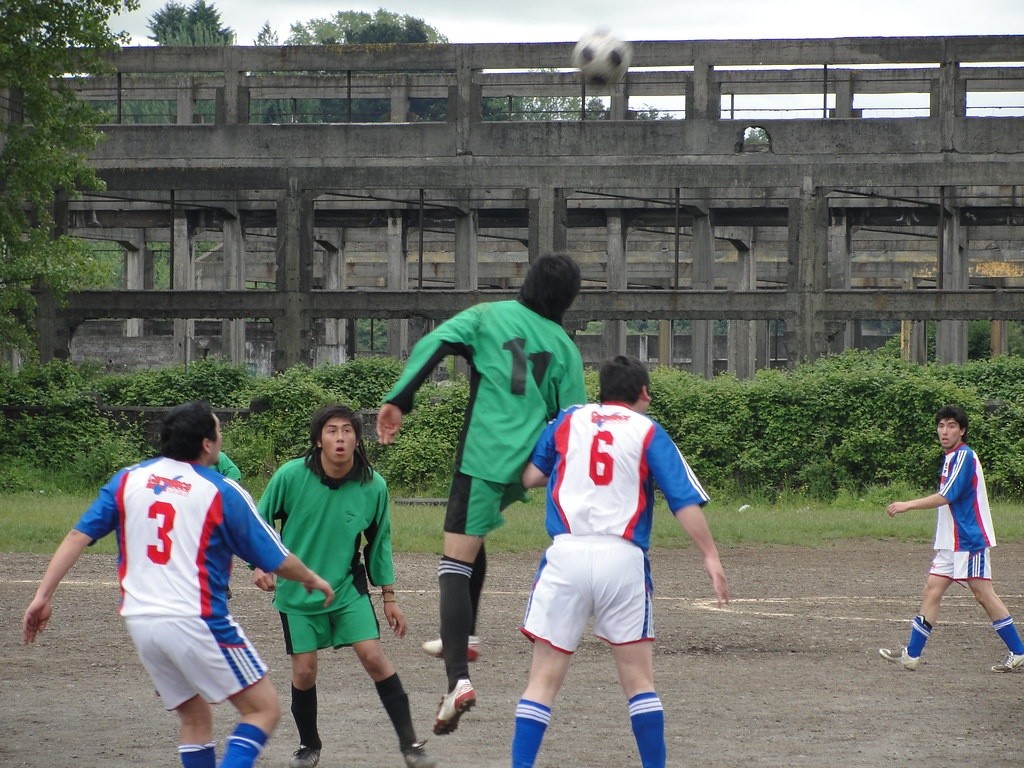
[{"x1": 572, "y1": 25, "x2": 632, "y2": 88}]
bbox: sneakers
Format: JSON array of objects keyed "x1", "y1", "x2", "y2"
[
  {"x1": 422, "y1": 635, "x2": 481, "y2": 661},
  {"x1": 400, "y1": 739, "x2": 436, "y2": 768},
  {"x1": 878, "y1": 646, "x2": 921, "y2": 671},
  {"x1": 288, "y1": 744, "x2": 320, "y2": 768},
  {"x1": 432, "y1": 678, "x2": 477, "y2": 735},
  {"x1": 991, "y1": 651, "x2": 1024, "y2": 674}
]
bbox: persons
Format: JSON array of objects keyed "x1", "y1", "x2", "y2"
[
  {"x1": 879, "y1": 407, "x2": 1024, "y2": 673},
  {"x1": 512, "y1": 355, "x2": 730, "y2": 768},
  {"x1": 248, "y1": 404, "x2": 435, "y2": 768},
  {"x1": 23, "y1": 403, "x2": 334, "y2": 768},
  {"x1": 376, "y1": 251, "x2": 588, "y2": 735}
]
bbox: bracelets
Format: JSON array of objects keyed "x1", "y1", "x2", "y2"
[
  {"x1": 381, "y1": 589, "x2": 394, "y2": 595},
  {"x1": 382, "y1": 598, "x2": 396, "y2": 604}
]
